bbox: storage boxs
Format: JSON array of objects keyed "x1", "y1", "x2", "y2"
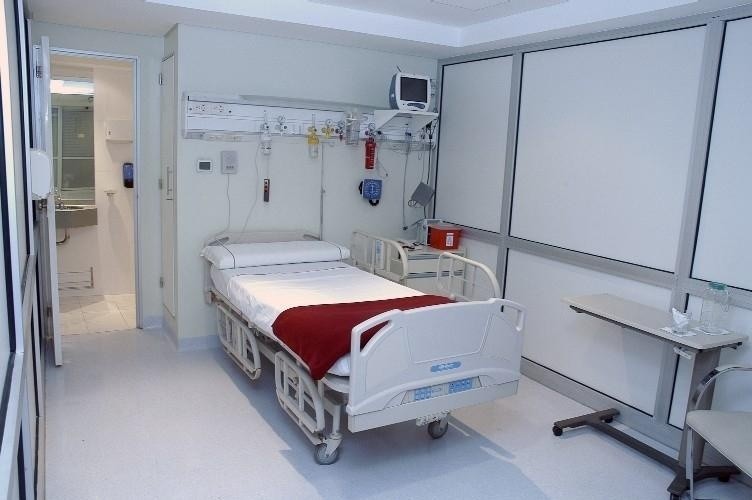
[{"x1": 428, "y1": 223, "x2": 463, "y2": 251}]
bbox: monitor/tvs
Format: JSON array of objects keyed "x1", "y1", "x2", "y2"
[{"x1": 389, "y1": 72, "x2": 432, "y2": 112}]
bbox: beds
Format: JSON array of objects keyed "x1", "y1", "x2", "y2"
[{"x1": 199, "y1": 231, "x2": 528, "y2": 466}]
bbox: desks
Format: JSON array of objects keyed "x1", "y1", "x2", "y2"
[{"x1": 551, "y1": 291, "x2": 749, "y2": 500}]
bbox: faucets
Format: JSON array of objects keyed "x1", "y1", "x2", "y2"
[{"x1": 60, "y1": 202, "x2": 65, "y2": 210}]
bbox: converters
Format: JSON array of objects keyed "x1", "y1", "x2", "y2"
[{"x1": 410, "y1": 182, "x2": 435, "y2": 206}]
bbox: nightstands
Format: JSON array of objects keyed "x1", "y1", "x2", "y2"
[{"x1": 390, "y1": 242, "x2": 465, "y2": 300}]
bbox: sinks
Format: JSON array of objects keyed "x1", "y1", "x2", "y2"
[{"x1": 55, "y1": 204, "x2": 97, "y2": 228}]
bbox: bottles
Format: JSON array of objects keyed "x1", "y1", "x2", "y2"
[
  {"x1": 699, "y1": 281, "x2": 730, "y2": 335},
  {"x1": 122, "y1": 162, "x2": 133, "y2": 188}
]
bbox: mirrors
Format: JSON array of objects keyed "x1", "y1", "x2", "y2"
[{"x1": 50, "y1": 78, "x2": 95, "y2": 202}]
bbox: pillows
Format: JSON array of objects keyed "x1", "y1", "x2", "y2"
[{"x1": 201, "y1": 235, "x2": 352, "y2": 271}]
